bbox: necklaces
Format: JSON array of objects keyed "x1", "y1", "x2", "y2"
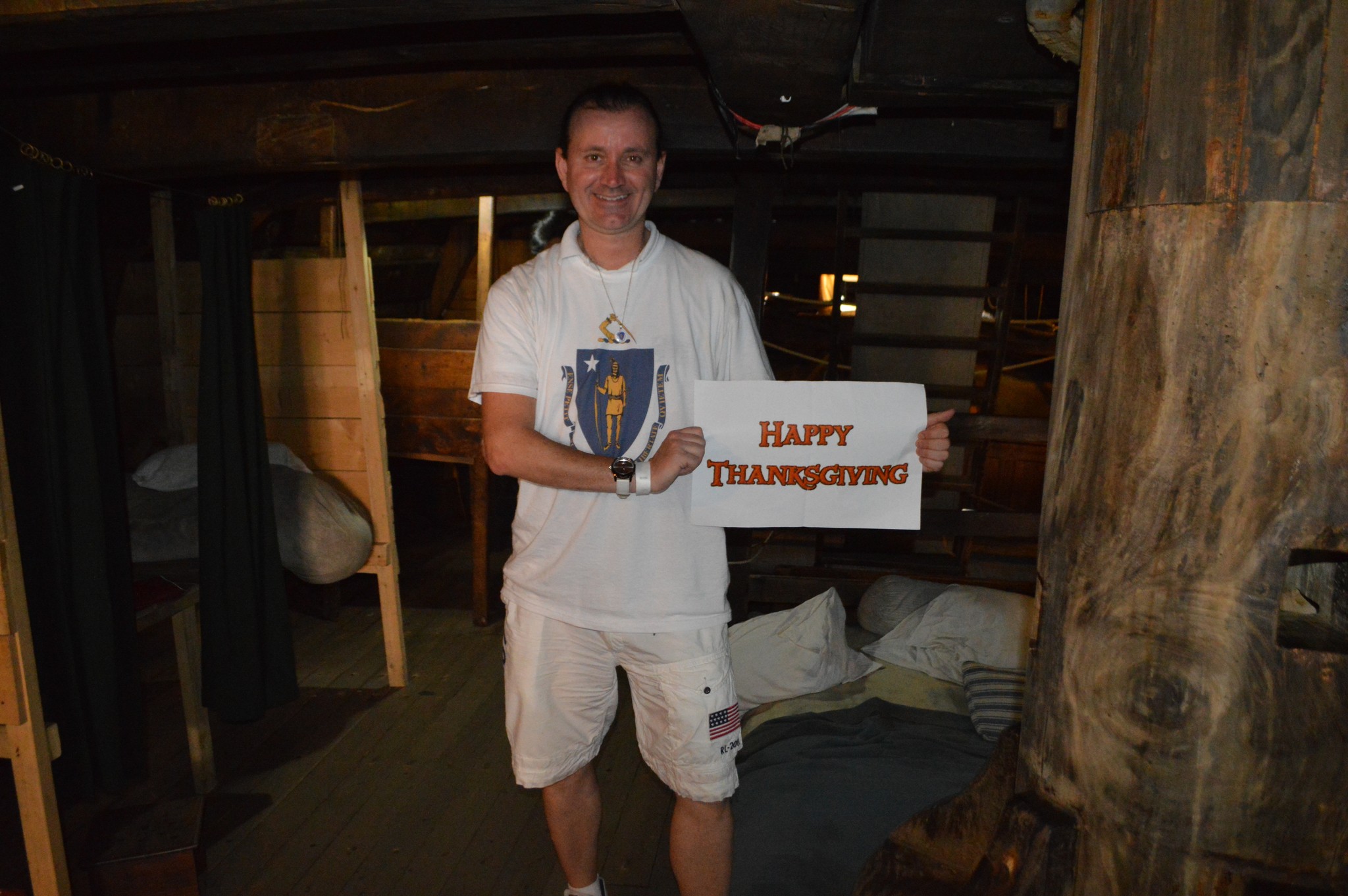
[{"x1": 580, "y1": 228, "x2": 645, "y2": 343}]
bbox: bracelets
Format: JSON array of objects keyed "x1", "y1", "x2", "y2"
[{"x1": 635, "y1": 461, "x2": 651, "y2": 496}]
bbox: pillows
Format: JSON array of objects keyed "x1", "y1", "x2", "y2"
[
  {"x1": 727, "y1": 585, "x2": 885, "y2": 721},
  {"x1": 857, "y1": 574, "x2": 951, "y2": 635},
  {"x1": 961, "y1": 660, "x2": 1026, "y2": 742},
  {"x1": 859, "y1": 584, "x2": 1036, "y2": 688}
]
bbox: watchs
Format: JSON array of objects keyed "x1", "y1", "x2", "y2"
[{"x1": 608, "y1": 457, "x2": 635, "y2": 499}]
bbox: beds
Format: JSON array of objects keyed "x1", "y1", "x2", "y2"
[{"x1": 724, "y1": 503, "x2": 1040, "y2": 896}]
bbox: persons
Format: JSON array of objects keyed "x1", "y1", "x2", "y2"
[{"x1": 466, "y1": 80, "x2": 956, "y2": 896}]
